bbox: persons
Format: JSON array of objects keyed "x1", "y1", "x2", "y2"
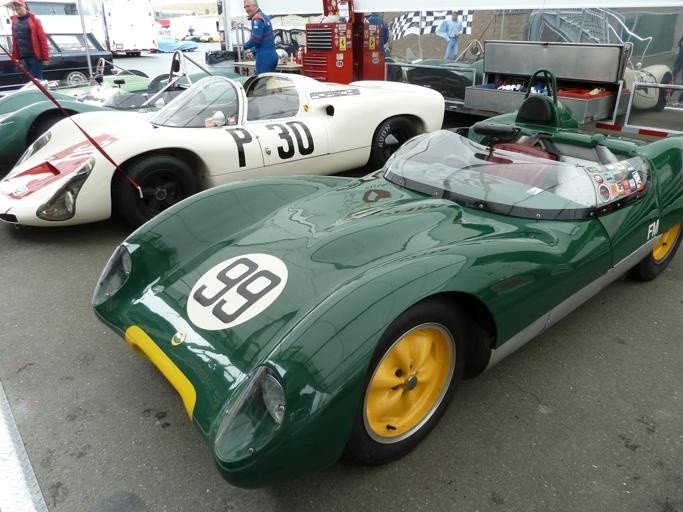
[
  {"x1": 366, "y1": 12, "x2": 389, "y2": 55},
  {"x1": 443, "y1": 12, "x2": 465, "y2": 60},
  {"x1": 240, "y1": 0, "x2": 279, "y2": 76},
  {"x1": 9, "y1": 0, "x2": 50, "y2": 81},
  {"x1": 671, "y1": 36, "x2": 682, "y2": 82}
]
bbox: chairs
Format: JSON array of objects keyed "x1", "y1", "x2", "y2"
[{"x1": 483, "y1": 144, "x2": 555, "y2": 186}]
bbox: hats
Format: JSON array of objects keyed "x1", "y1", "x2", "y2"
[{"x1": 7, "y1": 0, "x2": 27, "y2": 9}]
